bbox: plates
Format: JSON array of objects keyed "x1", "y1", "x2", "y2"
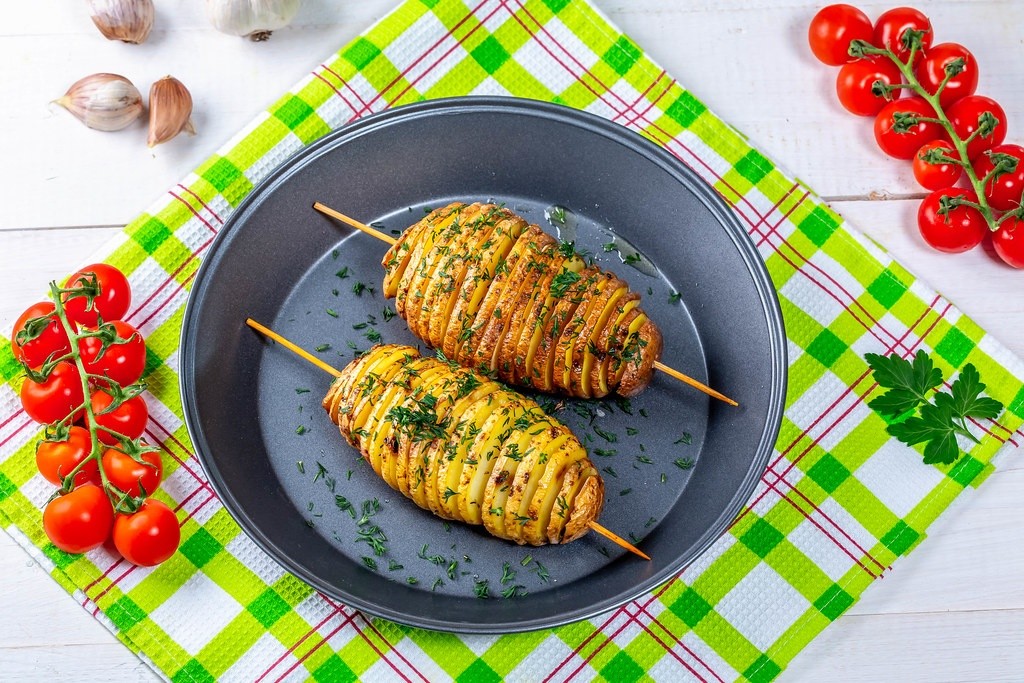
[{"x1": 176, "y1": 94, "x2": 786, "y2": 636}]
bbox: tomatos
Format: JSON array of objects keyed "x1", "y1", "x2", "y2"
[
  {"x1": 809, "y1": 6, "x2": 1024, "y2": 271},
  {"x1": 11, "y1": 262, "x2": 182, "y2": 566}
]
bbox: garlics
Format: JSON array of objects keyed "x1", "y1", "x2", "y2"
[{"x1": 45, "y1": 0, "x2": 298, "y2": 148}]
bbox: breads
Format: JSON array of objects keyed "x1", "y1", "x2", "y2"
[{"x1": 324, "y1": 199, "x2": 660, "y2": 544}]
